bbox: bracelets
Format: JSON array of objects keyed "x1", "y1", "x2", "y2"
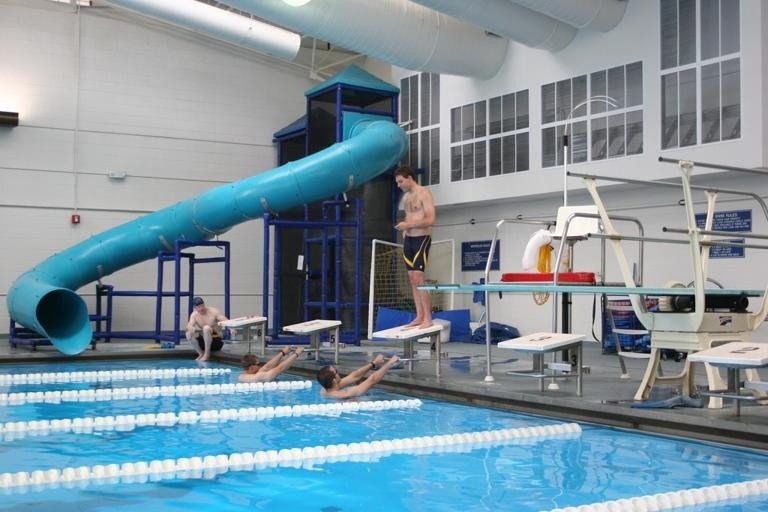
[
  {"x1": 294, "y1": 352, "x2": 299, "y2": 358},
  {"x1": 280, "y1": 350, "x2": 285, "y2": 356},
  {"x1": 370, "y1": 361, "x2": 376, "y2": 368}
]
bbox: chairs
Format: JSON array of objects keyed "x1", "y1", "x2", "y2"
[{"x1": 550, "y1": 205, "x2": 599, "y2": 272}]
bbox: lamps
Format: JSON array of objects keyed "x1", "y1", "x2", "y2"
[{"x1": 0, "y1": 111, "x2": 18, "y2": 126}]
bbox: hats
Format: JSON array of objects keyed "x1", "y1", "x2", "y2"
[{"x1": 191, "y1": 296, "x2": 204, "y2": 306}]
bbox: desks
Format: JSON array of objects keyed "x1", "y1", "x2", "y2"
[{"x1": 634, "y1": 331, "x2": 768, "y2": 409}]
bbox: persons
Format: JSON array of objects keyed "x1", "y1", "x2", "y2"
[
  {"x1": 316, "y1": 352, "x2": 402, "y2": 399},
  {"x1": 185, "y1": 296, "x2": 238, "y2": 361},
  {"x1": 391, "y1": 165, "x2": 437, "y2": 331},
  {"x1": 238, "y1": 344, "x2": 305, "y2": 386}
]
